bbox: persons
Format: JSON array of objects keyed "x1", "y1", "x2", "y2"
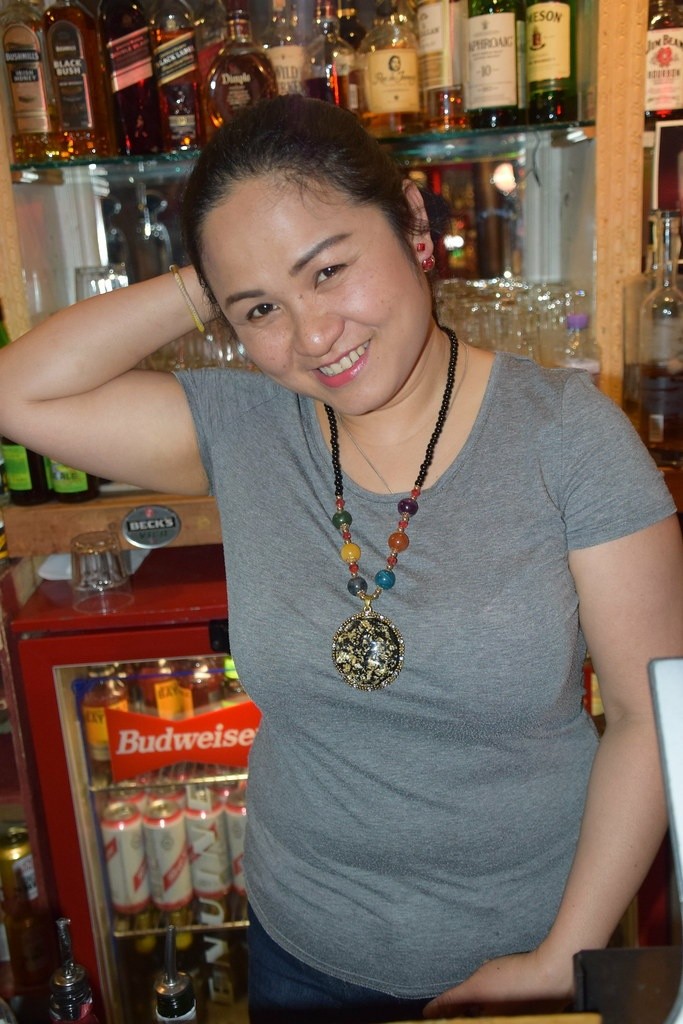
[{"x1": 0, "y1": 96, "x2": 683, "y2": 1024}]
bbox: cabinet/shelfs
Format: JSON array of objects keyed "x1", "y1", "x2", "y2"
[{"x1": 0, "y1": 0, "x2": 651, "y2": 563}]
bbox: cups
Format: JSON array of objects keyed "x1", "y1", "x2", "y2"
[
  {"x1": 72, "y1": 267, "x2": 586, "y2": 373},
  {"x1": 68, "y1": 530, "x2": 134, "y2": 616}
]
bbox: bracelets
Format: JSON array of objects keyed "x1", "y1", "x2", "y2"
[{"x1": 169, "y1": 265, "x2": 208, "y2": 333}]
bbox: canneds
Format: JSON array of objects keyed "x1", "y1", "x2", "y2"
[
  {"x1": 0, "y1": 827, "x2": 47, "y2": 984},
  {"x1": 100, "y1": 768, "x2": 250, "y2": 915}
]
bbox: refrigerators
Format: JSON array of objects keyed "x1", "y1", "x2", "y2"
[{"x1": 12, "y1": 545, "x2": 263, "y2": 1024}]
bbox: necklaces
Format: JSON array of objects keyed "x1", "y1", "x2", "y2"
[
  {"x1": 336, "y1": 343, "x2": 471, "y2": 492},
  {"x1": 324, "y1": 324, "x2": 460, "y2": 692}
]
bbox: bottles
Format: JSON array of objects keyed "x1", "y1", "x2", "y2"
[
  {"x1": 625, "y1": 207, "x2": 683, "y2": 470},
  {"x1": 641, "y1": 0, "x2": 682, "y2": 125},
  {"x1": 554, "y1": 292, "x2": 603, "y2": 388},
  {"x1": 0, "y1": 0, "x2": 583, "y2": 166},
  {"x1": 1, "y1": 439, "x2": 94, "y2": 507},
  {"x1": 76, "y1": 655, "x2": 242, "y2": 781}
]
